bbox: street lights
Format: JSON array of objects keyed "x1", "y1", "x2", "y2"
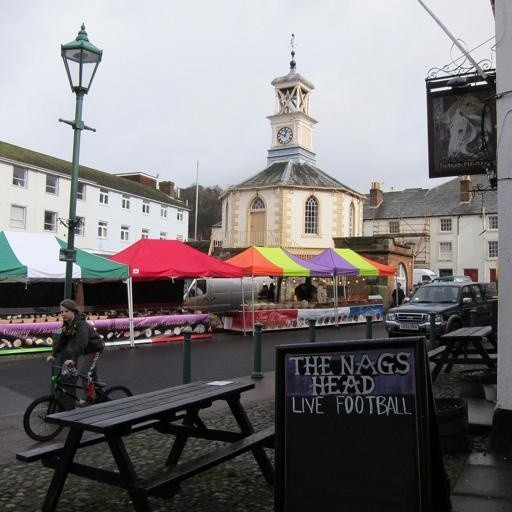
[{"x1": 56, "y1": 21, "x2": 100, "y2": 349}]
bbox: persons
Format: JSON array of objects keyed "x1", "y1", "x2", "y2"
[
  {"x1": 392, "y1": 282, "x2": 405, "y2": 306},
  {"x1": 268, "y1": 282, "x2": 276, "y2": 302},
  {"x1": 45, "y1": 299, "x2": 105, "y2": 407},
  {"x1": 258, "y1": 284, "x2": 268, "y2": 300},
  {"x1": 415, "y1": 282, "x2": 422, "y2": 293}
]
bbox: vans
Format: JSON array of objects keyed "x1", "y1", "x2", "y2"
[
  {"x1": 183, "y1": 277, "x2": 273, "y2": 312},
  {"x1": 407, "y1": 268, "x2": 436, "y2": 286}
]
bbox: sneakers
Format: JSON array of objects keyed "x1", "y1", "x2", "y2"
[{"x1": 76, "y1": 399, "x2": 92, "y2": 407}]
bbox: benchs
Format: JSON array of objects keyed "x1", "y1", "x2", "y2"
[
  {"x1": 427, "y1": 345, "x2": 446, "y2": 361},
  {"x1": 14, "y1": 412, "x2": 185, "y2": 464},
  {"x1": 131, "y1": 424, "x2": 275, "y2": 494}
]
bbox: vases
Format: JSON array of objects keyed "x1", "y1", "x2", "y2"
[{"x1": 483, "y1": 384, "x2": 497, "y2": 401}]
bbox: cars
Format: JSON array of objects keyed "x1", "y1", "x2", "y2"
[{"x1": 430, "y1": 275, "x2": 473, "y2": 283}]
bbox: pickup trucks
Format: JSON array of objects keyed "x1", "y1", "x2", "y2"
[{"x1": 385, "y1": 282, "x2": 496, "y2": 338}]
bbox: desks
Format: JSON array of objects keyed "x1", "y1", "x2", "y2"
[
  {"x1": 430, "y1": 326, "x2": 497, "y2": 383},
  {"x1": 0, "y1": 312, "x2": 213, "y2": 351},
  {"x1": 231, "y1": 303, "x2": 385, "y2": 331},
  {"x1": 42, "y1": 379, "x2": 275, "y2": 511}
]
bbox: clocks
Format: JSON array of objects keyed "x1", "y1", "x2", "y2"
[{"x1": 276, "y1": 126, "x2": 293, "y2": 145}]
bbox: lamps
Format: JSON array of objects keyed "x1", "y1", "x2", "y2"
[{"x1": 482, "y1": 165, "x2": 497, "y2": 188}]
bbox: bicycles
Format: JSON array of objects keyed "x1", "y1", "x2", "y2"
[{"x1": 18, "y1": 374, "x2": 135, "y2": 444}]
